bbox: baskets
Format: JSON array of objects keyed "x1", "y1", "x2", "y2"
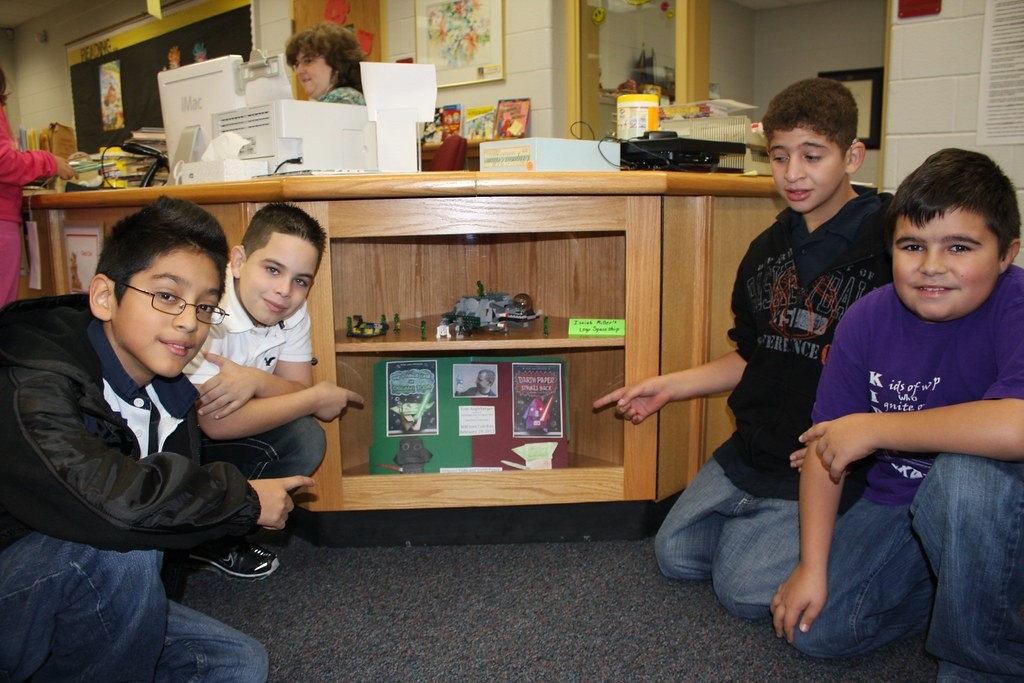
[{"x1": 661, "y1": 115, "x2": 774, "y2": 170}]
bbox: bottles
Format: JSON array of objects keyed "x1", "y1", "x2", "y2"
[{"x1": 617, "y1": 94, "x2": 659, "y2": 141}]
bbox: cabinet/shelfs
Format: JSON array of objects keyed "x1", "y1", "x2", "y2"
[{"x1": 247, "y1": 193, "x2": 670, "y2": 511}]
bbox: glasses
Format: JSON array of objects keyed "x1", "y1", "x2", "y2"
[{"x1": 112, "y1": 274, "x2": 229, "y2": 326}]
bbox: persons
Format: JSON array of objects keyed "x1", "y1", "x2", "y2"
[
  {"x1": 591, "y1": 79, "x2": 936, "y2": 627},
  {"x1": 152, "y1": 200, "x2": 367, "y2": 580},
  {"x1": 767, "y1": 147, "x2": 1023, "y2": 683},
  {"x1": 0, "y1": 195, "x2": 319, "y2": 683},
  {"x1": 455, "y1": 369, "x2": 496, "y2": 396},
  {"x1": 0, "y1": 68, "x2": 79, "y2": 308},
  {"x1": 282, "y1": 21, "x2": 370, "y2": 108}
]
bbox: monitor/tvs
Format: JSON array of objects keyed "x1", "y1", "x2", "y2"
[{"x1": 157, "y1": 54, "x2": 243, "y2": 169}]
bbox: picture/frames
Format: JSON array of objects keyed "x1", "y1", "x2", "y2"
[
  {"x1": 817, "y1": 65, "x2": 887, "y2": 153},
  {"x1": 412, "y1": 0, "x2": 508, "y2": 92}
]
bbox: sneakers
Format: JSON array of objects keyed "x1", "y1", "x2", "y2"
[{"x1": 188, "y1": 537, "x2": 279, "y2": 580}]
